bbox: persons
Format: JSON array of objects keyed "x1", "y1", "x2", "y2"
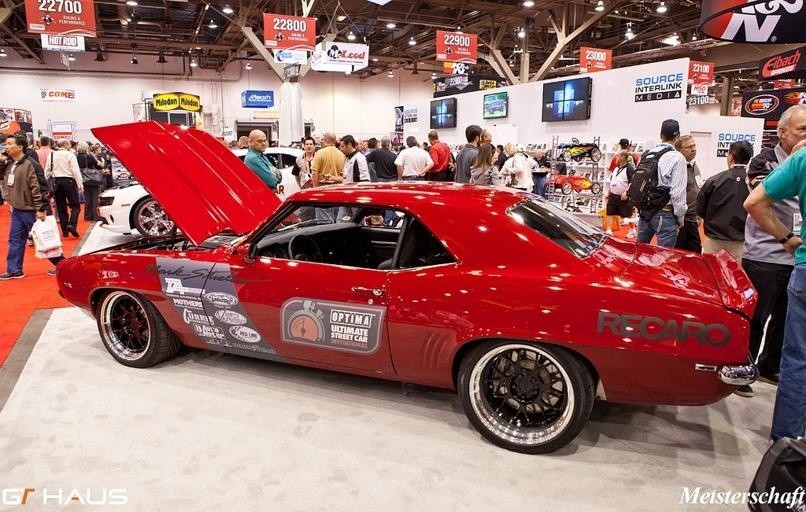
[
  {"x1": 603, "y1": 103, "x2": 806, "y2": 441},
  {"x1": 244, "y1": 120, "x2": 553, "y2": 196},
  {"x1": 1, "y1": 131, "x2": 112, "y2": 284}
]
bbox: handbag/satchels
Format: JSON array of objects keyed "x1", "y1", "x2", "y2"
[
  {"x1": 29, "y1": 214, "x2": 64, "y2": 260},
  {"x1": 80, "y1": 168, "x2": 104, "y2": 186},
  {"x1": 46, "y1": 175, "x2": 56, "y2": 193}
]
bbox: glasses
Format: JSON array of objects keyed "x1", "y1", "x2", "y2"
[{"x1": 723, "y1": 152, "x2": 732, "y2": 159}]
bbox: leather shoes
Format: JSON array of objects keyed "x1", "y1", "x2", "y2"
[
  {"x1": 66, "y1": 224, "x2": 79, "y2": 238},
  {"x1": 62, "y1": 231, "x2": 68, "y2": 237}
]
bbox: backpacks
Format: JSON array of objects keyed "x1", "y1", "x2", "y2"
[{"x1": 626, "y1": 146, "x2": 676, "y2": 212}]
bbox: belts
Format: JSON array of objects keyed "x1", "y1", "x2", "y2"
[
  {"x1": 401, "y1": 175, "x2": 425, "y2": 179},
  {"x1": 662, "y1": 205, "x2": 674, "y2": 211}
]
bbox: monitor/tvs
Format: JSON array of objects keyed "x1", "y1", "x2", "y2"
[
  {"x1": 430, "y1": 97, "x2": 457, "y2": 129},
  {"x1": 542, "y1": 76, "x2": 592, "y2": 122},
  {"x1": 483, "y1": 91, "x2": 507, "y2": 119}
]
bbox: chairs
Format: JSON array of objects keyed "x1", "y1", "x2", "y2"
[{"x1": 372, "y1": 218, "x2": 421, "y2": 272}]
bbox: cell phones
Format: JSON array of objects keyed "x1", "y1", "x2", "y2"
[{"x1": 3, "y1": 151, "x2": 8, "y2": 156}]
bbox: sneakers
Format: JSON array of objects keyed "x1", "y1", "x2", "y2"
[
  {"x1": 733, "y1": 383, "x2": 754, "y2": 397},
  {"x1": 604, "y1": 214, "x2": 639, "y2": 240},
  {"x1": 0, "y1": 271, "x2": 25, "y2": 280},
  {"x1": 47, "y1": 267, "x2": 56, "y2": 275},
  {"x1": 754, "y1": 364, "x2": 780, "y2": 386}
]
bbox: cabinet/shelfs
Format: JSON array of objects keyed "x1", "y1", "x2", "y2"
[{"x1": 547, "y1": 142, "x2": 649, "y2": 211}]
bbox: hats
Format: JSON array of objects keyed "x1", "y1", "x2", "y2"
[
  {"x1": 618, "y1": 139, "x2": 629, "y2": 146},
  {"x1": 69, "y1": 139, "x2": 107, "y2": 156},
  {"x1": 660, "y1": 119, "x2": 679, "y2": 139}
]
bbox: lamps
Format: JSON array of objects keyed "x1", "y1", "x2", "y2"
[{"x1": 0, "y1": 0, "x2": 672, "y2": 83}]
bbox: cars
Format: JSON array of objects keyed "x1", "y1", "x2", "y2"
[
  {"x1": 55, "y1": 119, "x2": 760, "y2": 454},
  {"x1": 485, "y1": 99, "x2": 505, "y2": 115},
  {"x1": 96, "y1": 147, "x2": 318, "y2": 241},
  {"x1": 106, "y1": 155, "x2": 132, "y2": 185}
]
